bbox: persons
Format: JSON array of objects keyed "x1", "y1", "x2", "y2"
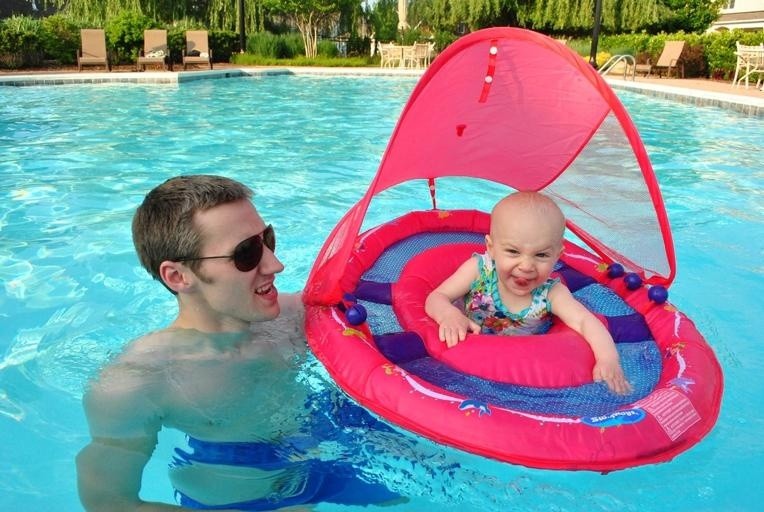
[
  {"x1": 76, "y1": 174, "x2": 417, "y2": 511},
  {"x1": 423, "y1": 191, "x2": 633, "y2": 395}
]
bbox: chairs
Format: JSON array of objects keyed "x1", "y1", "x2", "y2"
[
  {"x1": 376, "y1": 40, "x2": 431, "y2": 71},
  {"x1": 75, "y1": 28, "x2": 213, "y2": 71},
  {"x1": 730, "y1": 40, "x2": 764, "y2": 90},
  {"x1": 630, "y1": 40, "x2": 686, "y2": 79}
]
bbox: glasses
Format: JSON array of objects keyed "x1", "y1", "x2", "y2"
[{"x1": 172, "y1": 223, "x2": 277, "y2": 271}]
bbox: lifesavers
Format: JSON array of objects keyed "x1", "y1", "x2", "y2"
[{"x1": 302, "y1": 27, "x2": 725, "y2": 474}]
